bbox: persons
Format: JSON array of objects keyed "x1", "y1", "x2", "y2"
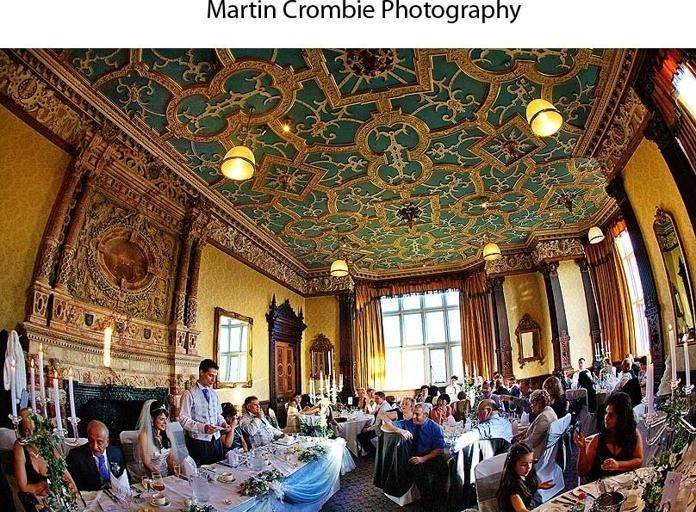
[
  {"x1": 286, "y1": 353, "x2": 647, "y2": 512},
  {"x1": 12, "y1": 407, "x2": 78, "y2": 512},
  {"x1": 239, "y1": 396, "x2": 285, "y2": 448},
  {"x1": 68, "y1": 420, "x2": 132, "y2": 491},
  {"x1": 178, "y1": 359, "x2": 231, "y2": 468},
  {"x1": 220, "y1": 402, "x2": 248, "y2": 461},
  {"x1": 132, "y1": 399, "x2": 178, "y2": 482}
]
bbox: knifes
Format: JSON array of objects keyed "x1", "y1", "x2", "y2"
[
  {"x1": 552, "y1": 488, "x2": 597, "y2": 506},
  {"x1": 103, "y1": 488, "x2": 121, "y2": 506}
]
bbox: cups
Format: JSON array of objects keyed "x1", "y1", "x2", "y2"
[
  {"x1": 152, "y1": 493, "x2": 165, "y2": 505},
  {"x1": 334, "y1": 406, "x2": 367, "y2": 422},
  {"x1": 221, "y1": 430, "x2": 326, "y2": 470},
  {"x1": 218, "y1": 471, "x2": 234, "y2": 482},
  {"x1": 594, "y1": 373, "x2": 619, "y2": 393},
  {"x1": 438, "y1": 417, "x2": 473, "y2": 443}
]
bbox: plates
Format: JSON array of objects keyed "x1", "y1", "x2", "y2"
[
  {"x1": 148, "y1": 499, "x2": 171, "y2": 508},
  {"x1": 78, "y1": 491, "x2": 98, "y2": 504},
  {"x1": 217, "y1": 477, "x2": 236, "y2": 483}
]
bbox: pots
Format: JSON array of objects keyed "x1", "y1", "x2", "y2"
[{"x1": 594, "y1": 490, "x2": 626, "y2": 512}]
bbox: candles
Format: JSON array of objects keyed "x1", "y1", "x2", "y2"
[
  {"x1": 465, "y1": 361, "x2": 476, "y2": 379},
  {"x1": 310, "y1": 370, "x2": 343, "y2": 397},
  {"x1": 647, "y1": 356, "x2": 653, "y2": 416},
  {"x1": 11, "y1": 343, "x2": 76, "y2": 430},
  {"x1": 684, "y1": 335, "x2": 690, "y2": 387},
  {"x1": 669, "y1": 324, "x2": 676, "y2": 381},
  {"x1": 595, "y1": 341, "x2": 610, "y2": 355}
]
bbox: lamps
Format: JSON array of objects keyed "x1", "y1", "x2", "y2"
[
  {"x1": 580, "y1": 194, "x2": 605, "y2": 244},
  {"x1": 220, "y1": 106, "x2": 256, "y2": 181},
  {"x1": 480, "y1": 208, "x2": 501, "y2": 261},
  {"x1": 331, "y1": 227, "x2": 348, "y2": 277},
  {"x1": 504, "y1": 61, "x2": 563, "y2": 137}
]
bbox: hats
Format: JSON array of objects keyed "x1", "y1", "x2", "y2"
[{"x1": 221, "y1": 402, "x2": 237, "y2": 416}]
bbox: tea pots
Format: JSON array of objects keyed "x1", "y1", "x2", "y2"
[{"x1": 187, "y1": 469, "x2": 213, "y2": 503}]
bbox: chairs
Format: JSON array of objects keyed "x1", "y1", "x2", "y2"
[
  {"x1": 1, "y1": 378, "x2": 369, "y2": 512},
  {"x1": 369, "y1": 380, "x2": 695, "y2": 511}
]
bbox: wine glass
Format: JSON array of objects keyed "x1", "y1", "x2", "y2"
[
  {"x1": 173, "y1": 463, "x2": 183, "y2": 482},
  {"x1": 139, "y1": 472, "x2": 165, "y2": 495},
  {"x1": 112, "y1": 483, "x2": 134, "y2": 510}
]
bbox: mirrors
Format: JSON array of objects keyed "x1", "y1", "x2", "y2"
[
  {"x1": 212, "y1": 307, "x2": 253, "y2": 388},
  {"x1": 654, "y1": 206, "x2": 696, "y2": 346},
  {"x1": 310, "y1": 333, "x2": 333, "y2": 379},
  {"x1": 515, "y1": 313, "x2": 544, "y2": 369}
]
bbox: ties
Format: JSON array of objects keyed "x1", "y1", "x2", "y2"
[
  {"x1": 202, "y1": 389, "x2": 209, "y2": 402},
  {"x1": 98, "y1": 455, "x2": 109, "y2": 479}
]
